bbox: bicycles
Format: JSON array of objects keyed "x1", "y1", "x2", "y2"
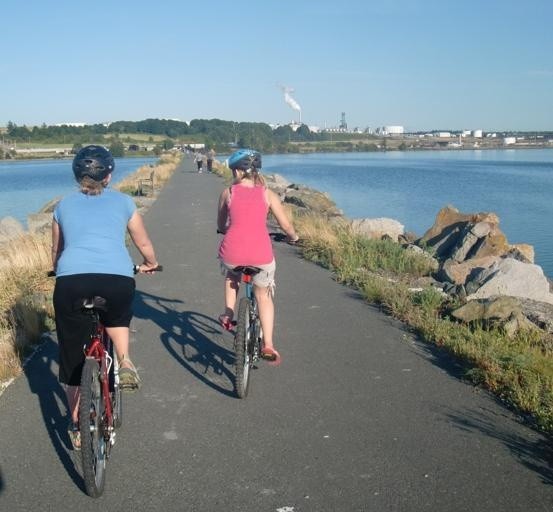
[
  {"x1": 215, "y1": 227, "x2": 301, "y2": 399},
  {"x1": 46, "y1": 264, "x2": 163, "y2": 499}
]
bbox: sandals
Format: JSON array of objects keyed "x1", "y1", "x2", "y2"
[
  {"x1": 118, "y1": 354, "x2": 142, "y2": 394},
  {"x1": 67, "y1": 421, "x2": 81, "y2": 451}
]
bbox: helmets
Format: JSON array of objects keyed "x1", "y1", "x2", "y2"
[
  {"x1": 229, "y1": 149, "x2": 262, "y2": 170},
  {"x1": 72, "y1": 145, "x2": 115, "y2": 184}
]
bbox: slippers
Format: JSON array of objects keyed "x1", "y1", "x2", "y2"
[
  {"x1": 218, "y1": 314, "x2": 233, "y2": 330},
  {"x1": 260, "y1": 346, "x2": 281, "y2": 366}
]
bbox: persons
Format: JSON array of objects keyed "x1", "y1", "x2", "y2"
[
  {"x1": 217, "y1": 147, "x2": 298, "y2": 369},
  {"x1": 53, "y1": 145, "x2": 159, "y2": 452},
  {"x1": 193, "y1": 149, "x2": 215, "y2": 173}
]
